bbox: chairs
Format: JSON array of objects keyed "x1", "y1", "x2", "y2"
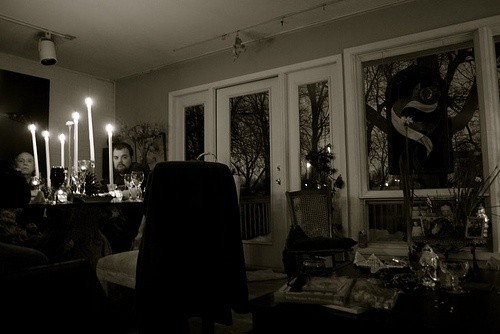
[
  {"x1": 95, "y1": 161, "x2": 248, "y2": 334},
  {"x1": 0, "y1": 240, "x2": 92, "y2": 334},
  {"x1": 285, "y1": 186, "x2": 353, "y2": 273}
]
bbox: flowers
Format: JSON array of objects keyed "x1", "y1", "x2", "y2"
[{"x1": 125, "y1": 119, "x2": 163, "y2": 163}]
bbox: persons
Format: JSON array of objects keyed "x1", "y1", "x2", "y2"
[
  {"x1": 4, "y1": 150, "x2": 49, "y2": 206},
  {"x1": 98, "y1": 142, "x2": 150, "y2": 253},
  {"x1": 411, "y1": 221, "x2": 422, "y2": 237}
]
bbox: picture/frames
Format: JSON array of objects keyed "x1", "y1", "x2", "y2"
[
  {"x1": 464, "y1": 215, "x2": 485, "y2": 239},
  {"x1": 410, "y1": 216, "x2": 425, "y2": 236}
]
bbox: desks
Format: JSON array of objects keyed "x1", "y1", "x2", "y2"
[
  {"x1": 16, "y1": 198, "x2": 145, "y2": 273},
  {"x1": 244, "y1": 266, "x2": 500, "y2": 334}
]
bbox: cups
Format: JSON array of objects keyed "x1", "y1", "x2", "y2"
[{"x1": 42, "y1": 186, "x2": 56, "y2": 205}]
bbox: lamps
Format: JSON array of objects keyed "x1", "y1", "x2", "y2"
[{"x1": 37, "y1": 30, "x2": 57, "y2": 66}]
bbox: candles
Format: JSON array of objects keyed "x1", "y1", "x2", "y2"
[
  {"x1": 27, "y1": 123, "x2": 40, "y2": 179},
  {"x1": 41, "y1": 129, "x2": 51, "y2": 187},
  {"x1": 58, "y1": 133, "x2": 67, "y2": 168},
  {"x1": 72, "y1": 112, "x2": 80, "y2": 171},
  {"x1": 85, "y1": 97, "x2": 95, "y2": 161},
  {"x1": 105, "y1": 123, "x2": 115, "y2": 185}
]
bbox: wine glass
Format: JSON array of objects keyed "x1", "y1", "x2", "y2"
[
  {"x1": 440, "y1": 259, "x2": 470, "y2": 294},
  {"x1": 123, "y1": 173, "x2": 133, "y2": 199},
  {"x1": 131, "y1": 171, "x2": 144, "y2": 200},
  {"x1": 71, "y1": 166, "x2": 86, "y2": 193}
]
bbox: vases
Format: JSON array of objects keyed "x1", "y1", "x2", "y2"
[{"x1": 137, "y1": 152, "x2": 151, "y2": 178}]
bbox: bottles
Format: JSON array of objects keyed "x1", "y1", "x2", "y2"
[
  {"x1": 57, "y1": 168, "x2": 71, "y2": 202},
  {"x1": 359, "y1": 227, "x2": 368, "y2": 248}
]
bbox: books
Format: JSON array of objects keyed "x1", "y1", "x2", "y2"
[{"x1": 274, "y1": 276, "x2": 398, "y2": 315}]
